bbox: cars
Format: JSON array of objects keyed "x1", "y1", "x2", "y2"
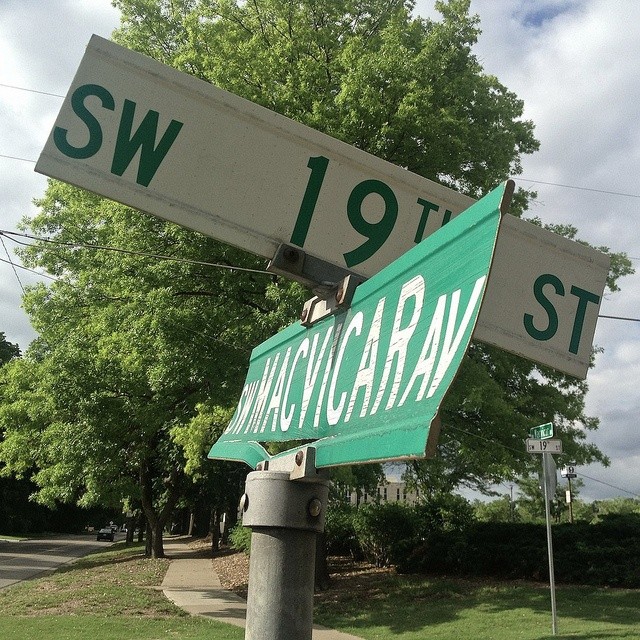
[
  {"x1": 97, "y1": 529, "x2": 113, "y2": 542},
  {"x1": 110, "y1": 525, "x2": 118, "y2": 532}
]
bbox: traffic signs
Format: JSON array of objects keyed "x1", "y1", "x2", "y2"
[
  {"x1": 526, "y1": 439, "x2": 561, "y2": 454},
  {"x1": 208, "y1": 180, "x2": 515, "y2": 473},
  {"x1": 530, "y1": 422, "x2": 555, "y2": 440},
  {"x1": 561, "y1": 463, "x2": 576, "y2": 475},
  {"x1": 34, "y1": 34, "x2": 610, "y2": 381}
]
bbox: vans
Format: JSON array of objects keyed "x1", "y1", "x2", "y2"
[{"x1": 171, "y1": 522, "x2": 186, "y2": 534}]
